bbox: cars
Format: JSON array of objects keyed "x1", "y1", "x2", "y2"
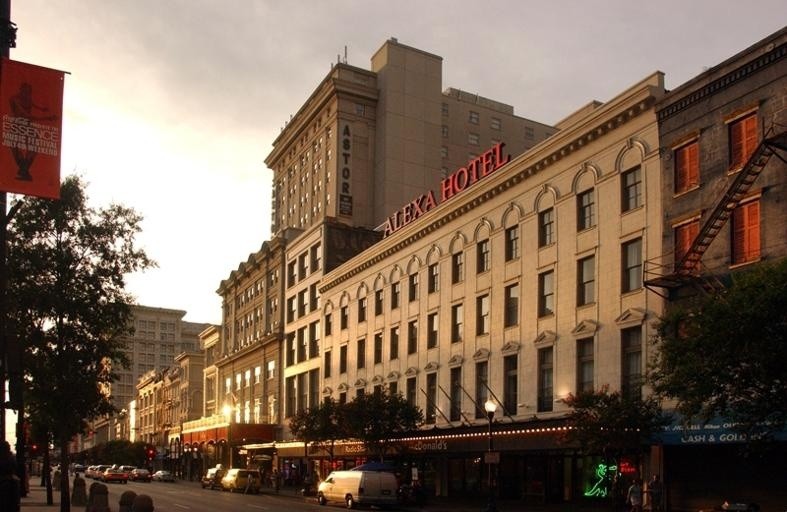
[
  {"x1": 57, "y1": 463, "x2": 84, "y2": 473},
  {"x1": 84, "y1": 464, "x2": 135, "y2": 485},
  {"x1": 129, "y1": 469, "x2": 151, "y2": 483},
  {"x1": 151, "y1": 470, "x2": 173, "y2": 482}
]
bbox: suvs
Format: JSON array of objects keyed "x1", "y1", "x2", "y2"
[{"x1": 200, "y1": 467, "x2": 225, "y2": 490}]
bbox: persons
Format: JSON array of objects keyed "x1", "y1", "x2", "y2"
[
  {"x1": 0, "y1": 439, "x2": 26, "y2": 512},
  {"x1": 7, "y1": 82, "x2": 56, "y2": 181},
  {"x1": 244, "y1": 462, "x2": 306, "y2": 497},
  {"x1": 625, "y1": 479, "x2": 643, "y2": 512},
  {"x1": 646, "y1": 474, "x2": 663, "y2": 512}
]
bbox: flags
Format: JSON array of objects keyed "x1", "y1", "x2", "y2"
[{"x1": 0, "y1": 55, "x2": 63, "y2": 201}]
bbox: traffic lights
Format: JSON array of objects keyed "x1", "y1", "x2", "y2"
[
  {"x1": 30, "y1": 442, "x2": 38, "y2": 456},
  {"x1": 148, "y1": 448, "x2": 153, "y2": 462}
]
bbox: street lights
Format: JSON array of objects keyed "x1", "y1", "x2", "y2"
[{"x1": 483, "y1": 393, "x2": 498, "y2": 512}]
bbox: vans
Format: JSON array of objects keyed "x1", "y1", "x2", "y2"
[
  {"x1": 316, "y1": 470, "x2": 399, "y2": 511},
  {"x1": 220, "y1": 469, "x2": 261, "y2": 493}
]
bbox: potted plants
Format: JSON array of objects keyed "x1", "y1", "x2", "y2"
[{"x1": 51, "y1": 472, "x2": 153, "y2": 511}]
